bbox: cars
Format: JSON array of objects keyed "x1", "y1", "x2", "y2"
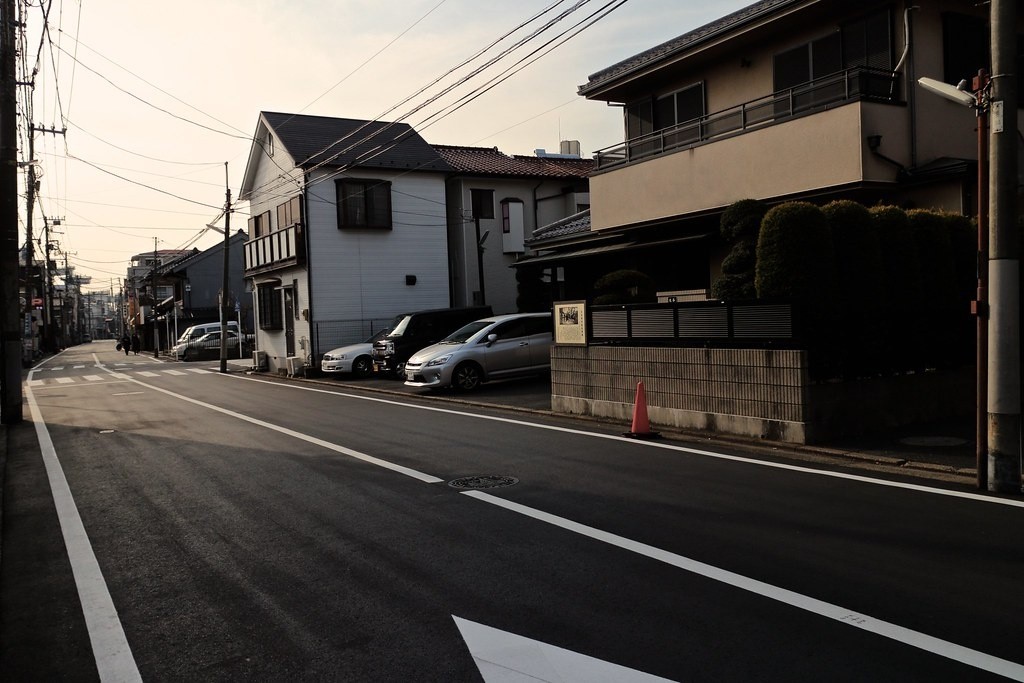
[
  {"x1": 321, "y1": 326, "x2": 388, "y2": 381},
  {"x1": 83, "y1": 333, "x2": 92, "y2": 343},
  {"x1": 170, "y1": 330, "x2": 255, "y2": 362}
]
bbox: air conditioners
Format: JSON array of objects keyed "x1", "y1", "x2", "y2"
[
  {"x1": 286, "y1": 357, "x2": 302, "y2": 375},
  {"x1": 252, "y1": 351, "x2": 266, "y2": 367}
]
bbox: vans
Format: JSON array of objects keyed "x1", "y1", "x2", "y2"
[
  {"x1": 371, "y1": 305, "x2": 494, "y2": 382},
  {"x1": 176, "y1": 320, "x2": 239, "y2": 345}
]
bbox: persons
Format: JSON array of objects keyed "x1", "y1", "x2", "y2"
[{"x1": 121, "y1": 334, "x2": 140, "y2": 356}]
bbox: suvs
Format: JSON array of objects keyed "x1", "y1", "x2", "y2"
[{"x1": 403, "y1": 312, "x2": 555, "y2": 397}]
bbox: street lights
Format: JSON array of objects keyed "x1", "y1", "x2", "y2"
[{"x1": 916, "y1": 68, "x2": 988, "y2": 493}]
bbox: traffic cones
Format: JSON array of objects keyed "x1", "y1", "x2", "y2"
[{"x1": 620, "y1": 380, "x2": 663, "y2": 441}]
